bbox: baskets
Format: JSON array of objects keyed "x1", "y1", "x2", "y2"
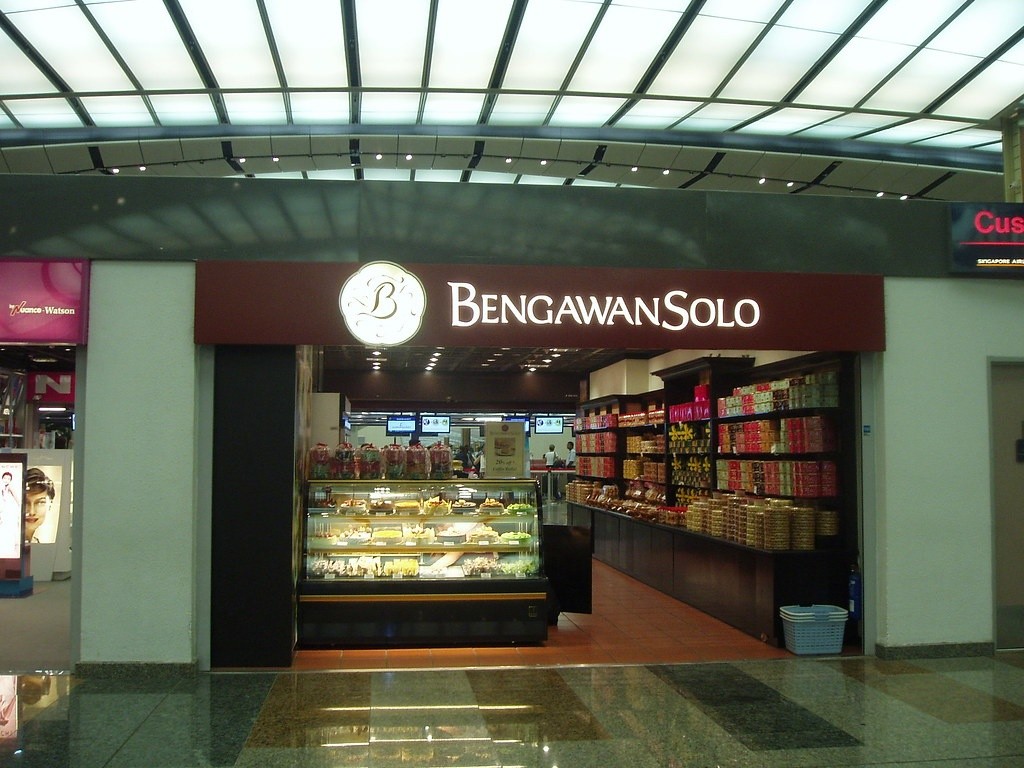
[{"x1": 780, "y1": 605, "x2": 848, "y2": 654}]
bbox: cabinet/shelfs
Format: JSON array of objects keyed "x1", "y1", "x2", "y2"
[
  {"x1": 295, "y1": 477, "x2": 549, "y2": 650},
  {"x1": 566, "y1": 351, "x2": 857, "y2": 648}
]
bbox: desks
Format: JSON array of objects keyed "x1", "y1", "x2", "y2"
[{"x1": 531, "y1": 468, "x2": 575, "y2": 501}]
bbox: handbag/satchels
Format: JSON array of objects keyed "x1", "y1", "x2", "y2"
[
  {"x1": 553, "y1": 452, "x2": 562, "y2": 467},
  {"x1": 467, "y1": 454, "x2": 472, "y2": 468}
]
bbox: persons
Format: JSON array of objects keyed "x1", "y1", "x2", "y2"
[
  {"x1": 409, "y1": 439, "x2": 421, "y2": 448},
  {"x1": 0, "y1": 473, "x2": 21, "y2": 558},
  {"x1": 454, "y1": 443, "x2": 486, "y2": 478},
  {"x1": 543, "y1": 444, "x2": 559, "y2": 468},
  {"x1": 24, "y1": 468, "x2": 55, "y2": 544},
  {"x1": 565, "y1": 441, "x2": 576, "y2": 483}
]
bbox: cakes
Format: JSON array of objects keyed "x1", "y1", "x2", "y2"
[
  {"x1": 307, "y1": 532, "x2": 336, "y2": 547},
  {"x1": 424, "y1": 499, "x2": 449, "y2": 514},
  {"x1": 501, "y1": 531, "x2": 531, "y2": 544},
  {"x1": 339, "y1": 499, "x2": 367, "y2": 514},
  {"x1": 451, "y1": 499, "x2": 476, "y2": 513},
  {"x1": 369, "y1": 499, "x2": 393, "y2": 514},
  {"x1": 339, "y1": 531, "x2": 371, "y2": 543},
  {"x1": 479, "y1": 497, "x2": 505, "y2": 513},
  {"x1": 372, "y1": 530, "x2": 402, "y2": 544},
  {"x1": 471, "y1": 526, "x2": 499, "y2": 543},
  {"x1": 395, "y1": 500, "x2": 420, "y2": 513},
  {"x1": 308, "y1": 498, "x2": 337, "y2": 513},
  {"x1": 435, "y1": 531, "x2": 466, "y2": 543},
  {"x1": 507, "y1": 503, "x2": 533, "y2": 514},
  {"x1": 404, "y1": 527, "x2": 432, "y2": 544}
]
